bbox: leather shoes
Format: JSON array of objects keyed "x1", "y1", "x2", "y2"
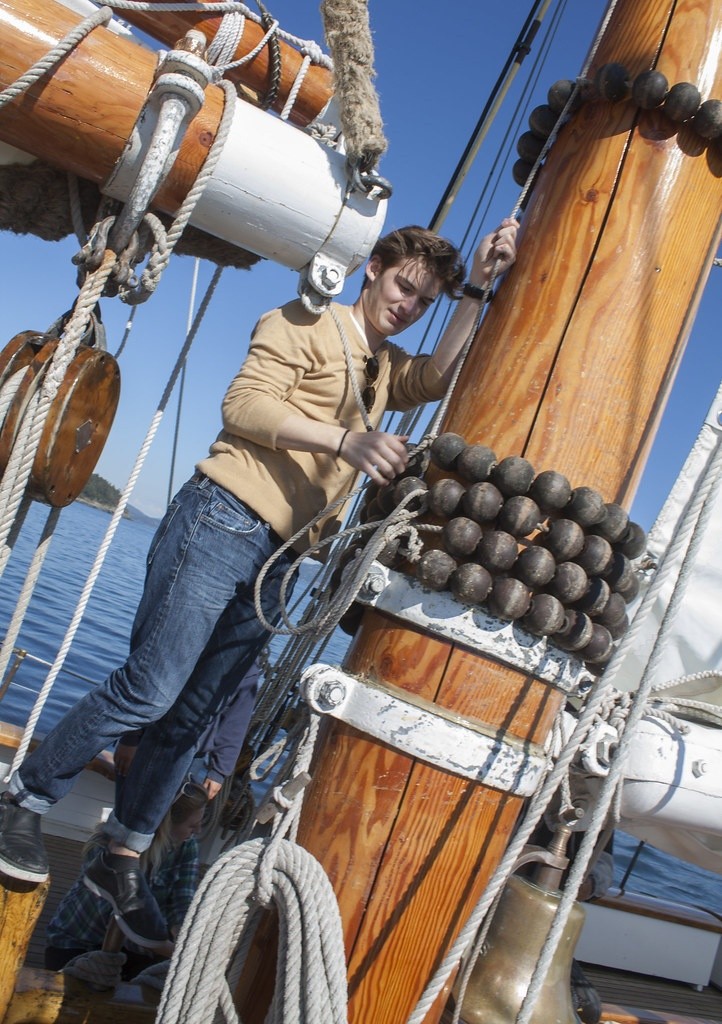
[
  {"x1": 83, "y1": 848, "x2": 171, "y2": 949},
  {"x1": 0, "y1": 792, "x2": 50, "y2": 883}
]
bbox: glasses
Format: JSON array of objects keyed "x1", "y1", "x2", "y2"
[
  {"x1": 172, "y1": 771, "x2": 197, "y2": 807},
  {"x1": 363, "y1": 355, "x2": 379, "y2": 415}
]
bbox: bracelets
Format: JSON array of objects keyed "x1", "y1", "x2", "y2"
[
  {"x1": 463, "y1": 282, "x2": 494, "y2": 304},
  {"x1": 336, "y1": 428, "x2": 352, "y2": 457}
]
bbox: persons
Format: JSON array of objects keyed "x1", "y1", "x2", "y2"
[
  {"x1": 43, "y1": 778, "x2": 209, "y2": 983},
  {"x1": 0, "y1": 213, "x2": 524, "y2": 952},
  {"x1": 112, "y1": 656, "x2": 260, "y2": 802},
  {"x1": 515, "y1": 800, "x2": 616, "y2": 903}
]
